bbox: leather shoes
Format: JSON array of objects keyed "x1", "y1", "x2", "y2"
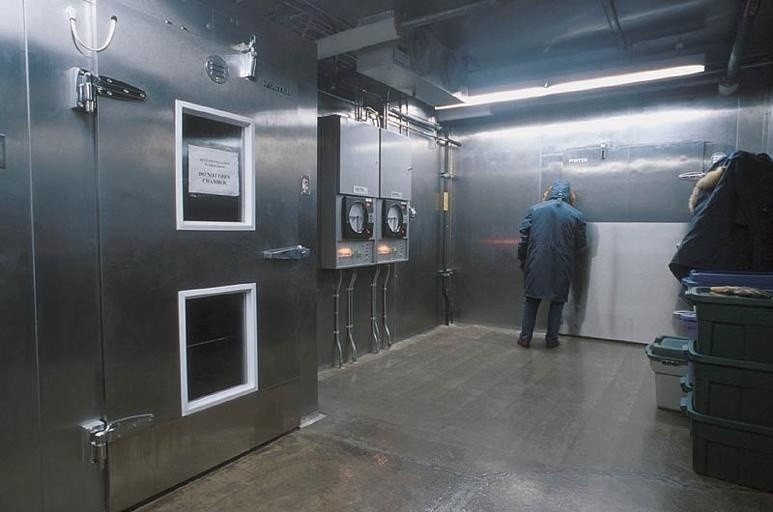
[
  {"x1": 517, "y1": 339, "x2": 529, "y2": 347},
  {"x1": 545, "y1": 339, "x2": 559, "y2": 348}
]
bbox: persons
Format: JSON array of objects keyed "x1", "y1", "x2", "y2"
[{"x1": 516, "y1": 179, "x2": 588, "y2": 349}]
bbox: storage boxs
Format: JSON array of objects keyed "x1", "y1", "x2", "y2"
[{"x1": 674, "y1": 270, "x2": 771, "y2": 491}]
